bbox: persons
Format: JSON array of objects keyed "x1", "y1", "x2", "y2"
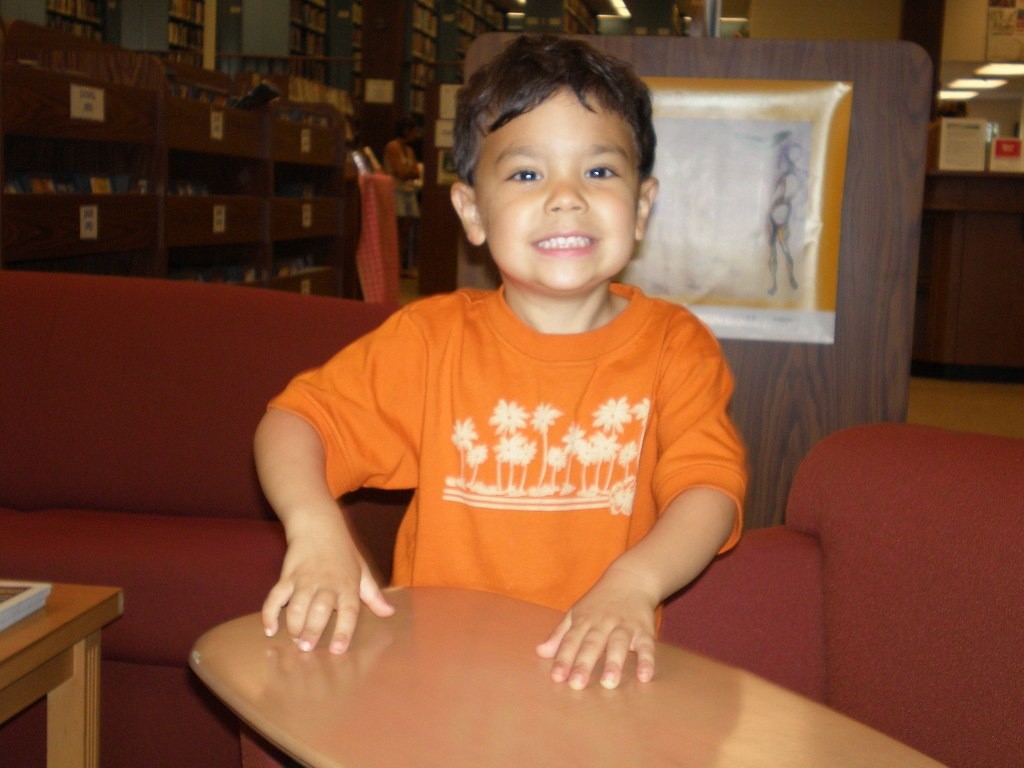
[
  {"x1": 252, "y1": 35, "x2": 754, "y2": 693},
  {"x1": 382, "y1": 110, "x2": 427, "y2": 277}
]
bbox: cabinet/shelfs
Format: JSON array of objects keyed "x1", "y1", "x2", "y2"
[{"x1": 1, "y1": 1, "x2": 684, "y2": 301}]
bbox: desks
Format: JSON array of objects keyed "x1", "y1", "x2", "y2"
[
  {"x1": 0, "y1": 579, "x2": 124, "y2": 768},
  {"x1": 190, "y1": 581, "x2": 949, "y2": 767}
]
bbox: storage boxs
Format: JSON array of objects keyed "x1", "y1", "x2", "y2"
[
  {"x1": 988, "y1": 137, "x2": 1023, "y2": 173},
  {"x1": 928, "y1": 117, "x2": 987, "y2": 173}
]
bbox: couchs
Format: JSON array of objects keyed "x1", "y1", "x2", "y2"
[
  {"x1": 656, "y1": 424, "x2": 1024, "y2": 768},
  {"x1": 0, "y1": 270, "x2": 412, "y2": 768}
]
